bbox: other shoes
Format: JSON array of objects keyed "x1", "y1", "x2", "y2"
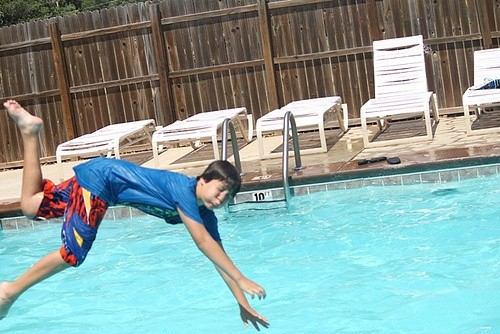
[
  {"x1": 358, "y1": 156, "x2": 386, "y2": 165},
  {"x1": 387, "y1": 156, "x2": 400, "y2": 164}
]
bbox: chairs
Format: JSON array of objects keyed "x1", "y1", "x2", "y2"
[
  {"x1": 462, "y1": 48, "x2": 500, "y2": 137},
  {"x1": 360, "y1": 35, "x2": 439, "y2": 148},
  {"x1": 152, "y1": 107, "x2": 254, "y2": 169},
  {"x1": 56, "y1": 119, "x2": 155, "y2": 180},
  {"x1": 256, "y1": 96, "x2": 349, "y2": 160}
]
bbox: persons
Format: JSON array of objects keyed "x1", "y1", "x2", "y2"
[{"x1": 0, "y1": 99, "x2": 270, "y2": 334}]
